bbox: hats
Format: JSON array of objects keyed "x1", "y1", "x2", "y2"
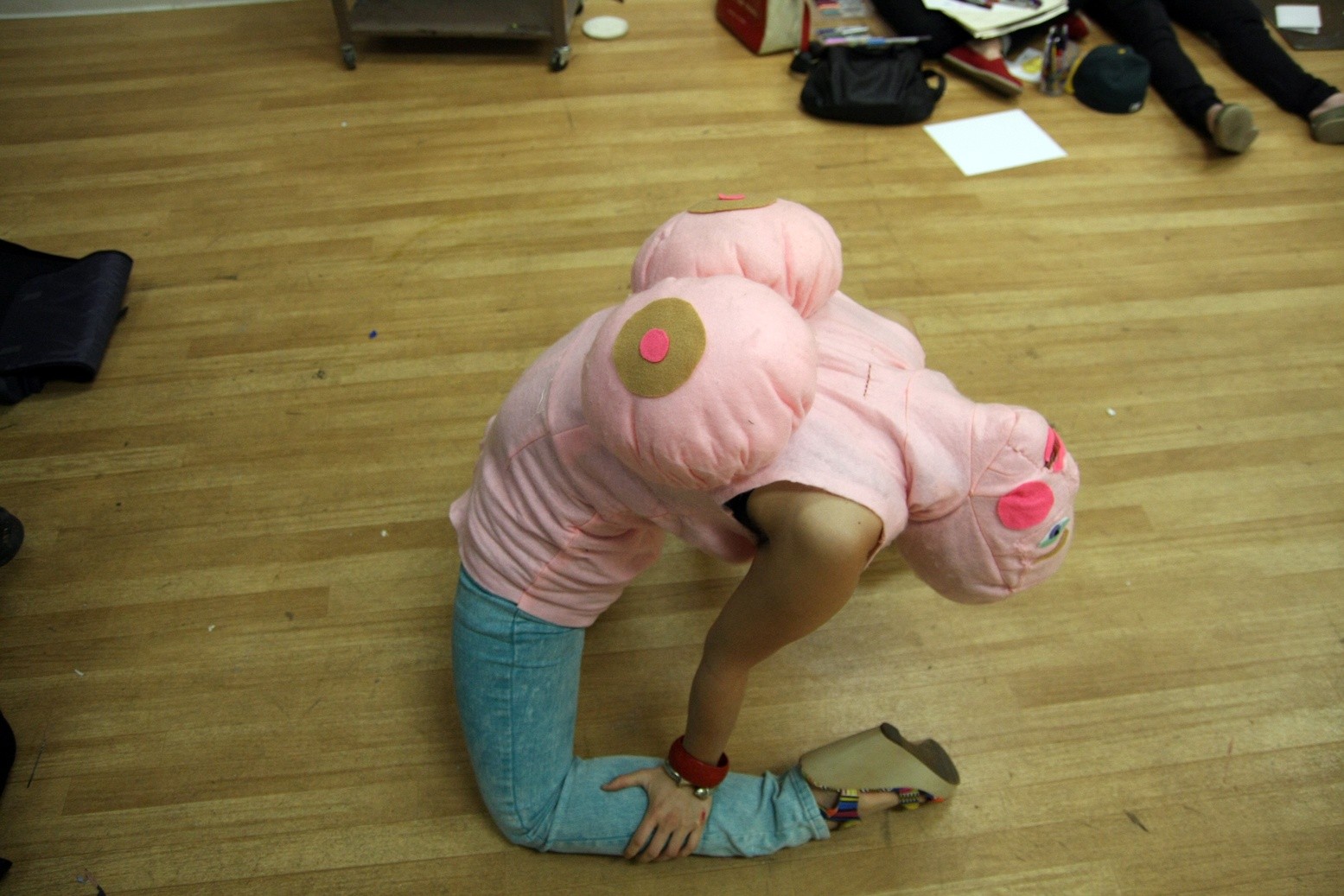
[{"x1": 1073, "y1": 45, "x2": 1150, "y2": 114}]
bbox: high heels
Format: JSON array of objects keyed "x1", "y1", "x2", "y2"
[{"x1": 795, "y1": 721, "x2": 961, "y2": 831}]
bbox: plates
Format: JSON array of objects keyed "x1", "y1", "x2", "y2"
[{"x1": 582, "y1": 16, "x2": 628, "y2": 39}]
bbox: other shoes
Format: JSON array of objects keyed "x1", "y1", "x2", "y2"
[
  {"x1": 1310, "y1": 105, "x2": 1344, "y2": 144},
  {"x1": 1213, "y1": 103, "x2": 1260, "y2": 154},
  {"x1": 943, "y1": 46, "x2": 1022, "y2": 95}
]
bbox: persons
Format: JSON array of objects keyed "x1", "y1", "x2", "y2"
[
  {"x1": 452, "y1": 186, "x2": 1080, "y2": 868},
  {"x1": 874, "y1": 0, "x2": 1344, "y2": 166}
]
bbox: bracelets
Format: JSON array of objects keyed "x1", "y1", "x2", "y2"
[{"x1": 662, "y1": 732, "x2": 730, "y2": 799}]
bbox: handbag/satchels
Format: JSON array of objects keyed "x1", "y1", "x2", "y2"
[{"x1": 790, "y1": 40, "x2": 947, "y2": 126}]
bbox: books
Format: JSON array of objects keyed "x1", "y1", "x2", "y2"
[{"x1": 923, "y1": 0, "x2": 1068, "y2": 40}]
